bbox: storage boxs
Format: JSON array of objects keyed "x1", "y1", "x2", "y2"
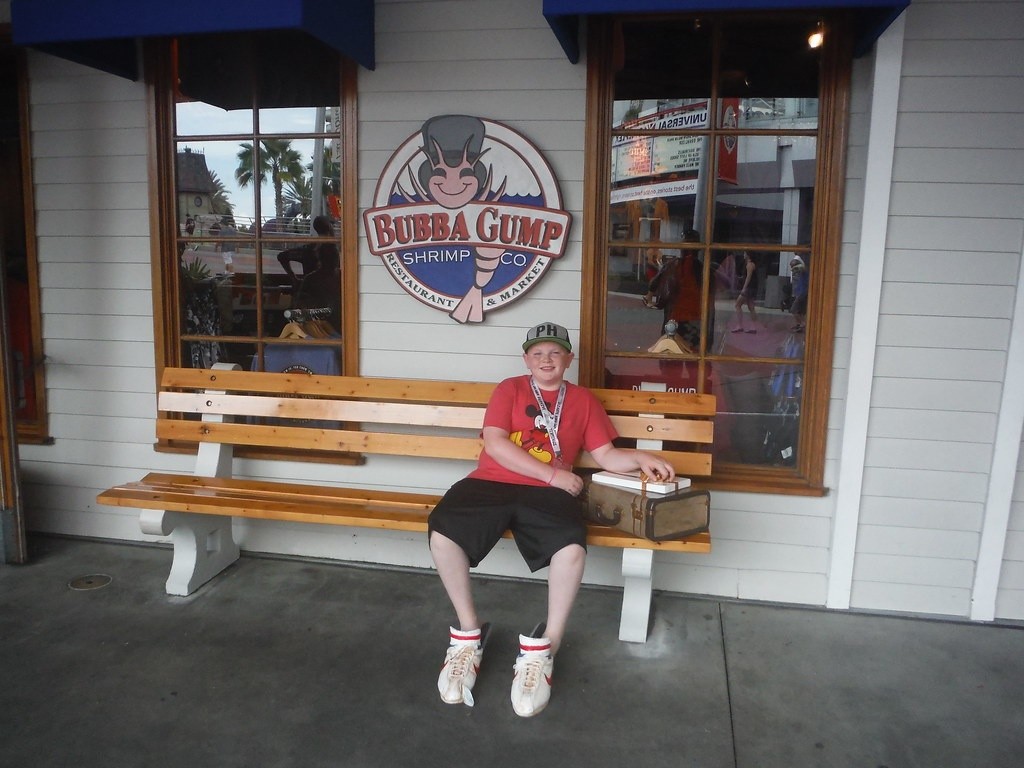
[{"x1": 590, "y1": 471, "x2": 692, "y2": 494}]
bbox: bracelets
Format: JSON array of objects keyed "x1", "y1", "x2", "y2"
[{"x1": 547, "y1": 466, "x2": 557, "y2": 487}]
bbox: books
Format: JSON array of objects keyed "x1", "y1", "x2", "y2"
[{"x1": 591, "y1": 474, "x2": 692, "y2": 494}]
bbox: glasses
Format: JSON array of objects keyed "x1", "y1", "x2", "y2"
[{"x1": 680, "y1": 231, "x2": 693, "y2": 237}]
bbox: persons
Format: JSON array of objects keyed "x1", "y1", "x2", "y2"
[
  {"x1": 644, "y1": 229, "x2": 714, "y2": 388},
  {"x1": 277, "y1": 216, "x2": 341, "y2": 311},
  {"x1": 209, "y1": 219, "x2": 237, "y2": 274},
  {"x1": 730, "y1": 251, "x2": 758, "y2": 335},
  {"x1": 788, "y1": 251, "x2": 810, "y2": 331},
  {"x1": 426, "y1": 323, "x2": 675, "y2": 718},
  {"x1": 183, "y1": 213, "x2": 199, "y2": 251}
]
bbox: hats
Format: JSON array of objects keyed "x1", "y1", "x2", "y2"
[{"x1": 522, "y1": 322, "x2": 571, "y2": 352}]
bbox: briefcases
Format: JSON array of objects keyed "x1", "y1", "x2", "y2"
[{"x1": 578, "y1": 480, "x2": 712, "y2": 541}]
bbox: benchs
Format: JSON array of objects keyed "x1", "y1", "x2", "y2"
[{"x1": 90, "y1": 358, "x2": 716, "y2": 646}]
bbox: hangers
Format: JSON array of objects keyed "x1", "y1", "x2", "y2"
[
  {"x1": 648, "y1": 320, "x2": 694, "y2": 356},
  {"x1": 278, "y1": 307, "x2": 335, "y2": 341}
]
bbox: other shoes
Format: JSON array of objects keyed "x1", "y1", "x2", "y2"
[
  {"x1": 188, "y1": 249, "x2": 193, "y2": 251},
  {"x1": 641, "y1": 295, "x2": 658, "y2": 309},
  {"x1": 791, "y1": 324, "x2": 805, "y2": 332},
  {"x1": 226, "y1": 271, "x2": 235, "y2": 276},
  {"x1": 194, "y1": 245, "x2": 199, "y2": 251},
  {"x1": 731, "y1": 328, "x2": 757, "y2": 335}
]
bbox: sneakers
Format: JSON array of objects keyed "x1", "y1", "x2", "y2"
[
  {"x1": 510, "y1": 623, "x2": 555, "y2": 717},
  {"x1": 437, "y1": 622, "x2": 493, "y2": 707}
]
